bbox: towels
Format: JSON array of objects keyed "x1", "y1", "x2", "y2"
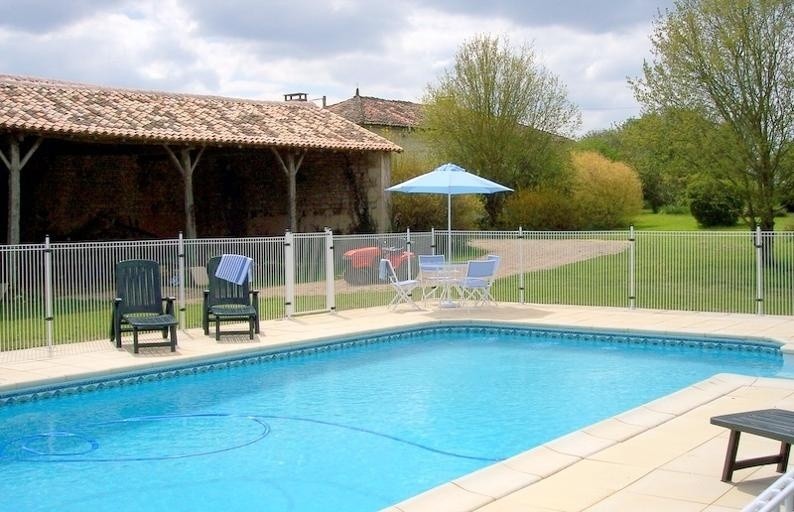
[{"x1": 215, "y1": 254, "x2": 254, "y2": 286}]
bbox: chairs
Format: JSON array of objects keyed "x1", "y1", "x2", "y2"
[
  {"x1": 380, "y1": 255, "x2": 501, "y2": 315},
  {"x1": 203, "y1": 256, "x2": 260, "y2": 339},
  {"x1": 110, "y1": 259, "x2": 177, "y2": 354}
]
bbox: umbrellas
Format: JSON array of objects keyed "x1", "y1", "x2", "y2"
[{"x1": 383, "y1": 163, "x2": 516, "y2": 301}]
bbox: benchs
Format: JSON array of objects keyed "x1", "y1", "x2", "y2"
[{"x1": 711, "y1": 409, "x2": 794, "y2": 482}]
